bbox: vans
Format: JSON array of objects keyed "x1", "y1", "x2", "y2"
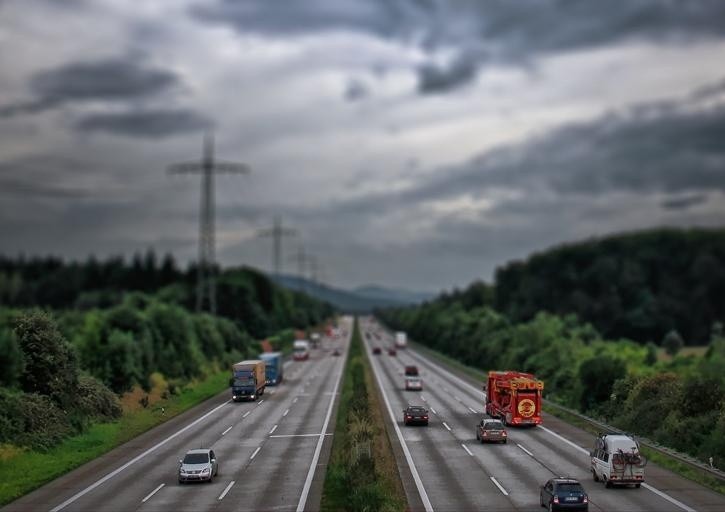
[
  {"x1": 177, "y1": 447, "x2": 219, "y2": 484},
  {"x1": 589, "y1": 432, "x2": 646, "y2": 489}
]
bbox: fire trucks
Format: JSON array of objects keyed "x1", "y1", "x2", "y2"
[{"x1": 482, "y1": 370, "x2": 545, "y2": 428}]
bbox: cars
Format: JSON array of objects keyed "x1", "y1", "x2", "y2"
[
  {"x1": 538, "y1": 477, "x2": 590, "y2": 511},
  {"x1": 309, "y1": 324, "x2": 347, "y2": 356},
  {"x1": 403, "y1": 407, "x2": 429, "y2": 427},
  {"x1": 405, "y1": 376, "x2": 422, "y2": 391},
  {"x1": 363, "y1": 323, "x2": 419, "y2": 377}
]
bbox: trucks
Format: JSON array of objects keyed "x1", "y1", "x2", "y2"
[
  {"x1": 292, "y1": 340, "x2": 309, "y2": 361},
  {"x1": 258, "y1": 351, "x2": 283, "y2": 386},
  {"x1": 229, "y1": 359, "x2": 266, "y2": 403}
]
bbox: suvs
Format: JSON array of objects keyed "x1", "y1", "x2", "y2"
[{"x1": 474, "y1": 417, "x2": 507, "y2": 444}]
